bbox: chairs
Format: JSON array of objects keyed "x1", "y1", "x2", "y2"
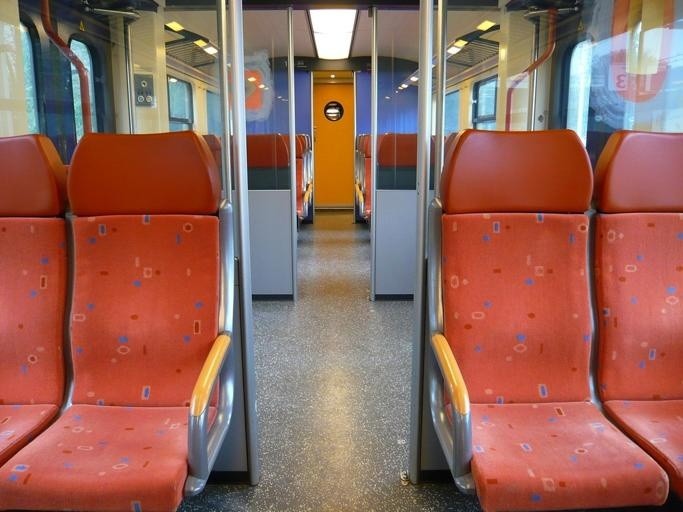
[
  {"x1": 202, "y1": 128, "x2": 312, "y2": 217},
  {"x1": 356, "y1": 131, "x2": 460, "y2": 229},
  {"x1": 443, "y1": 130, "x2": 683, "y2": 507},
  {"x1": 0, "y1": 131, "x2": 232, "y2": 511}
]
